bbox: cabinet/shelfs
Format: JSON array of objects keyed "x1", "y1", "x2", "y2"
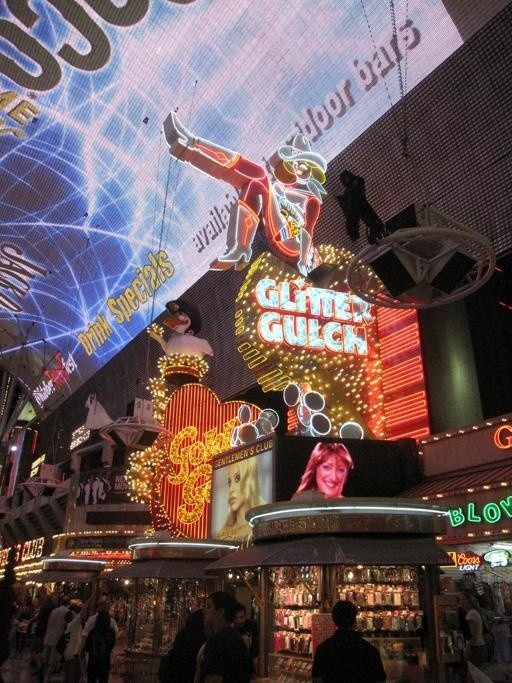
[{"x1": 267, "y1": 565, "x2": 425, "y2": 683}]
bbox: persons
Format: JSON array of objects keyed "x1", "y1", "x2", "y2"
[
  {"x1": 217, "y1": 456, "x2": 266, "y2": 540},
  {"x1": 290, "y1": 442, "x2": 354, "y2": 501},
  {"x1": 24, "y1": 592, "x2": 119, "y2": 682},
  {"x1": 441, "y1": 590, "x2": 488, "y2": 664},
  {"x1": 158, "y1": 590, "x2": 259, "y2": 683},
  {"x1": 311, "y1": 599, "x2": 387, "y2": 683}
]
